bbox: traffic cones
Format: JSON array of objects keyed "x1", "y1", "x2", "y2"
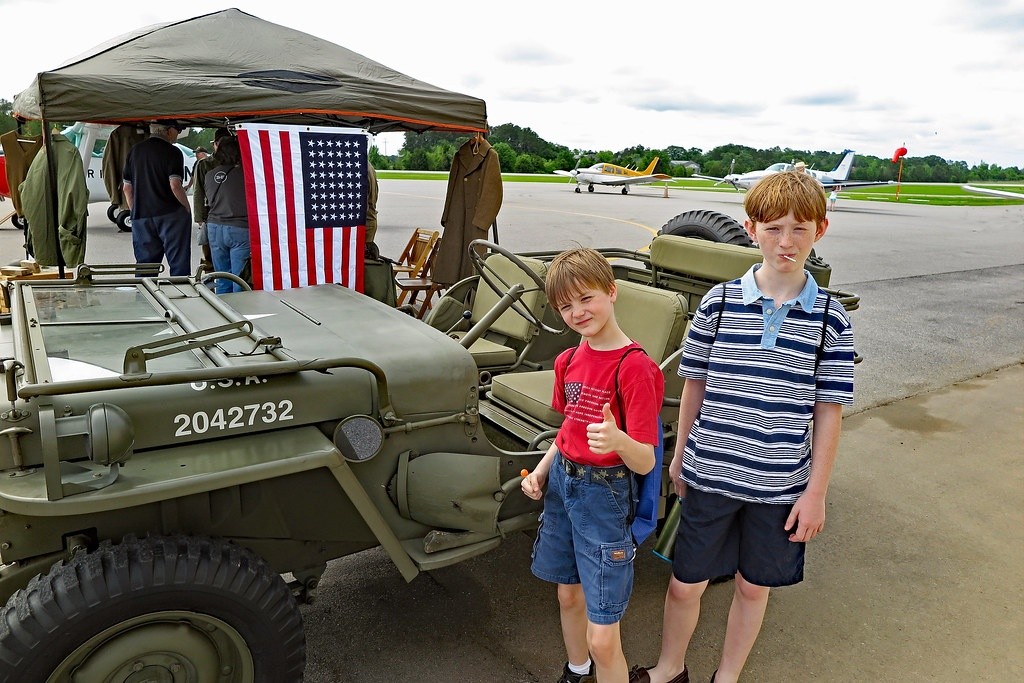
[{"x1": 662, "y1": 186, "x2": 670, "y2": 198}]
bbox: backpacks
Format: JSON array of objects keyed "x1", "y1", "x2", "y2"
[{"x1": 564, "y1": 343, "x2": 664, "y2": 548}]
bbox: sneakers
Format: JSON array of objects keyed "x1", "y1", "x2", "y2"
[{"x1": 557, "y1": 661, "x2": 597, "y2": 683}]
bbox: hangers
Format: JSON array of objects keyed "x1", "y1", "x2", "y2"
[
  {"x1": 14, "y1": 118, "x2": 41, "y2": 143},
  {"x1": 51, "y1": 121, "x2": 60, "y2": 135},
  {"x1": 469, "y1": 131, "x2": 484, "y2": 145}
]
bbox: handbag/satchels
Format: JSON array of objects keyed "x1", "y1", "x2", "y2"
[{"x1": 197, "y1": 222, "x2": 210, "y2": 246}]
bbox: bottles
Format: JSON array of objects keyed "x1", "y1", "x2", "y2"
[{"x1": 652, "y1": 498, "x2": 685, "y2": 562}]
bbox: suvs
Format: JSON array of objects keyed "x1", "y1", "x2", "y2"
[{"x1": 0, "y1": 200, "x2": 861, "y2": 683}]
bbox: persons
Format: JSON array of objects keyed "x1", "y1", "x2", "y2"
[
  {"x1": 829, "y1": 185, "x2": 841, "y2": 211},
  {"x1": 122, "y1": 118, "x2": 192, "y2": 277},
  {"x1": 628, "y1": 171, "x2": 856, "y2": 683},
  {"x1": 520, "y1": 239, "x2": 666, "y2": 683},
  {"x1": 794, "y1": 161, "x2": 810, "y2": 172},
  {"x1": 364, "y1": 148, "x2": 379, "y2": 243},
  {"x1": 183, "y1": 146, "x2": 209, "y2": 194},
  {"x1": 193, "y1": 128, "x2": 238, "y2": 285},
  {"x1": 204, "y1": 136, "x2": 251, "y2": 294}
]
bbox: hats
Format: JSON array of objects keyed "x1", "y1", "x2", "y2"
[
  {"x1": 214, "y1": 129, "x2": 235, "y2": 142},
  {"x1": 193, "y1": 146, "x2": 208, "y2": 154}
]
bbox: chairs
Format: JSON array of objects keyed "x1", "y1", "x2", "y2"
[
  {"x1": 392, "y1": 227, "x2": 439, "y2": 307},
  {"x1": 477, "y1": 279, "x2": 688, "y2": 451},
  {"x1": 394, "y1": 238, "x2": 449, "y2": 319},
  {"x1": 441, "y1": 254, "x2": 547, "y2": 372}
]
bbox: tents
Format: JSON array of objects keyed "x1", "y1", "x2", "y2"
[{"x1": 11, "y1": 5, "x2": 501, "y2": 281}]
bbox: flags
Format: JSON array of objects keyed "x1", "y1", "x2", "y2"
[{"x1": 229, "y1": 117, "x2": 370, "y2": 297}]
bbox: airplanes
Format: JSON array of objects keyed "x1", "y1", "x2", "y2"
[
  {"x1": 553, "y1": 156, "x2": 673, "y2": 195},
  {"x1": 691, "y1": 151, "x2": 895, "y2": 194}
]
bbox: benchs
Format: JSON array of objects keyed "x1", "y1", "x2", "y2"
[{"x1": 649, "y1": 234, "x2": 764, "y2": 349}]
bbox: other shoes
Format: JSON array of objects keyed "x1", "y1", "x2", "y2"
[{"x1": 628, "y1": 664, "x2": 718, "y2": 683}]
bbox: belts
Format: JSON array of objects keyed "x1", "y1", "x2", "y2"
[{"x1": 557, "y1": 450, "x2": 630, "y2": 495}]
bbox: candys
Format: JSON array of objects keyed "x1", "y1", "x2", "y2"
[{"x1": 521, "y1": 469, "x2": 528, "y2": 477}]
